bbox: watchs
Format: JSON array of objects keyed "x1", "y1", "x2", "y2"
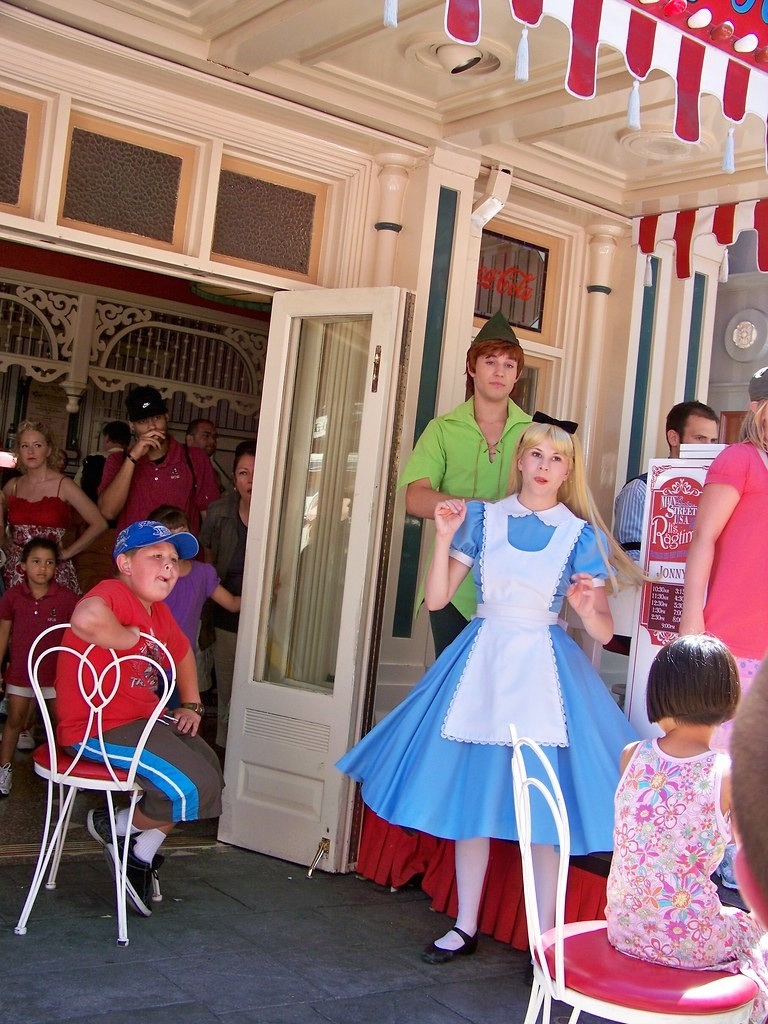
[{"x1": 181, "y1": 703, "x2": 205, "y2": 716}]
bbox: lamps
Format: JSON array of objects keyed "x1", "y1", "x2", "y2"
[{"x1": 437, "y1": 43, "x2": 481, "y2": 74}]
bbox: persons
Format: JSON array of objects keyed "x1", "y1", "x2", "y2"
[
  {"x1": 612, "y1": 397, "x2": 724, "y2": 654},
  {"x1": 53, "y1": 448, "x2": 68, "y2": 474},
  {"x1": 0, "y1": 420, "x2": 109, "y2": 749},
  {"x1": 405, "y1": 313, "x2": 539, "y2": 660},
  {"x1": 147, "y1": 505, "x2": 242, "y2": 712},
  {"x1": 0, "y1": 537, "x2": 81, "y2": 798},
  {"x1": 337, "y1": 409, "x2": 640, "y2": 989},
  {"x1": 687, "y1": 366, "x2": 768, "y2": 889},
  {"x1": 75, "y1": 379, "x2": 221, "y2": 700},
  {"x1": 200, "y1": 435, "x2": 258, "y2": 762},
  {"x1": 74, "y1": 420, "x2": 132, "y2": 533},
  {"x1": 54, "y1": 520, "x2": 226, "y2": 916},
  {"x1": 604, "y1": 635, "x2": 767, "y2": 1024}
]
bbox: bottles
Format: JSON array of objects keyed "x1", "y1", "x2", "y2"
[{"x1": 5, "y1": 423, "x2": 15, "y2": 449}]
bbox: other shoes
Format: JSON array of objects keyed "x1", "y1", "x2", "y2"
[
  {"x1": 421, "y1": 926, "x2": 479, "y2": 964},
  {"x1": 524, "y1": 956, "x2": 534, "y2": 985}
]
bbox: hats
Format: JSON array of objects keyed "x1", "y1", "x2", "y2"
[
  {"x1": 471, "y1": 309, "x2": 520, "y2": 347},
  {"x1": 114, "y1": 520, "x2": 199, "y2": 563},
  {"x1": 125, "y1": 385, "x2": 168, "y2": 421},
  {"x1": 749, "y1": 366, "x2": 768, "y2": 401}
]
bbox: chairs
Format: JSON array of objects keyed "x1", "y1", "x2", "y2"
[
  {"x1": 505, "y1": 722, "x2": 760, "y2": 1024},
  {"x1": 14, "y1": 625, "x2": 178, "y2": 948}
]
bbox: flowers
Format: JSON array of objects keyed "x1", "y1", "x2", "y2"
[{"x1": 732, "y1": 320, "x2": 758, "y2": 349}]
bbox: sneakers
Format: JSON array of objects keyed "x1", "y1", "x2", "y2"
[
  {"x1": 87, "y1": 806, "x2": 141, "y2": 847},
  {"x1": 103, "y1": 839, "x2": 165, "y2": 917},
  {"x1": 0, "y1": 761, "x2": 14, "y2": 796},
  {"x1": 15, "y1": 729, "x2": 35, "y2": 749}
]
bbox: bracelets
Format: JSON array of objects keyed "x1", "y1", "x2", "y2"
[{"x1": 127, "y1": 453, "x2": 138, "y2": 464}]
